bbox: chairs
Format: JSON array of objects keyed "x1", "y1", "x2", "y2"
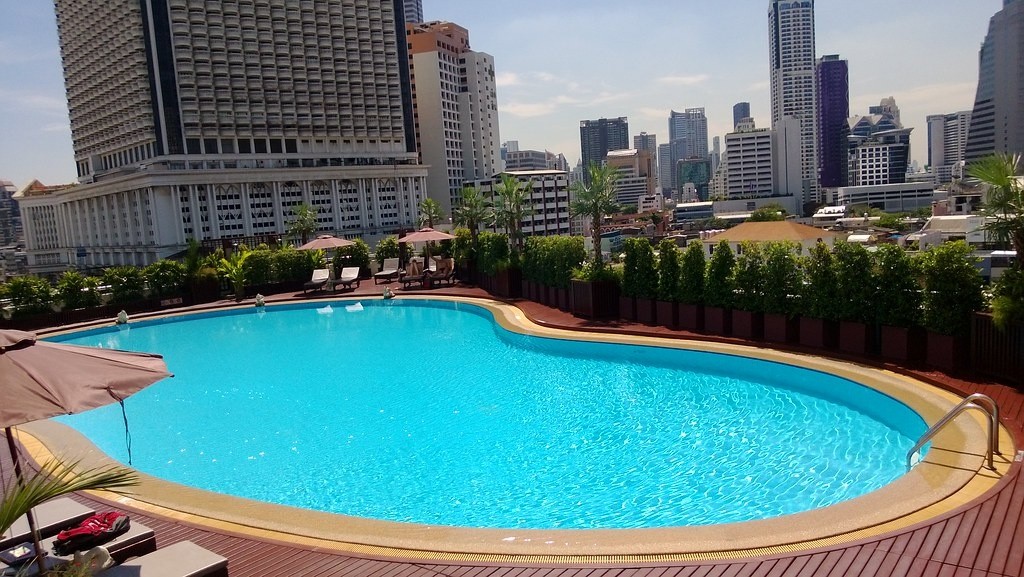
[
  {"x1": 398, "y1": 257, "x2": 425, "y2": 289},
  {"x1": 303, "y1": 269, "x2": 330, "y2": 296},
  {"x1": 429, "y1": 256, "x2": 442, "y2": 272},
  {"x1": 332, "y1": 267, "x2": 361, "y2": 295},
  {"x1": 374, "y1": 258, "x2": 399, "y2": 285},
  {"x1": 430, "y1": 257, "x2": 455, "y2": 285}
]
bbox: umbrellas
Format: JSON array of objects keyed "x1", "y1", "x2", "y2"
[
  {"x1": 397, "y1": 226, "x2": 459, "y2": 270},
  {"x1": 296, "y1": 234, "x2": 353, "y2": 270},
  {"x1": 0, "y1": 330, "x2": 175, "y2": 577}
]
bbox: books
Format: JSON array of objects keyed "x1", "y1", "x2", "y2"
[{"x1": 0, "y1": 542, "x2": 48, "y2": 568}]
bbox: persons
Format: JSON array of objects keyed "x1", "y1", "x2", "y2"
[
  {"x1": 428, "y1": 254, "x2": 452, "y2": 278},
  {"x1": 408, "y1": 259, "x2": 421, "y2": 278}
]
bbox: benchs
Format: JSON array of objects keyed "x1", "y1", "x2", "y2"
[
  {"x1": 93, "y1": 539, "x2": 229, "y2": 577},
  {"x1": 0, "y1": 497, "x2": 96, "y2": 551},
  {"x1": 0, "y1": 520, "x2": 157, "y2": 577}
]
